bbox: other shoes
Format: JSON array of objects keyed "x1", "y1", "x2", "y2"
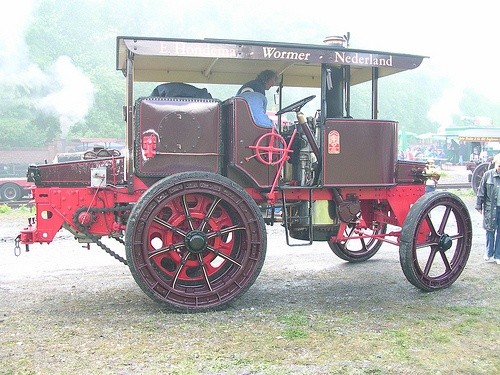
[{"x1": 485, "y1": 254, "x2": 500, "y2": 265}]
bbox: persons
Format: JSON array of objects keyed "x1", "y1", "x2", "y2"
[
  {"x1": 475, "y1": 154, "x2": 500, "y2": 266},
  {"x1": 238, "y1": 87, "x2": 284, "y2": 186},
  {"x1": 237, "y1": 70, "x2": 275, "y2": 96},
  {"x1": 401, "y1": 136, "x2": 471, "y2": 168}
]
